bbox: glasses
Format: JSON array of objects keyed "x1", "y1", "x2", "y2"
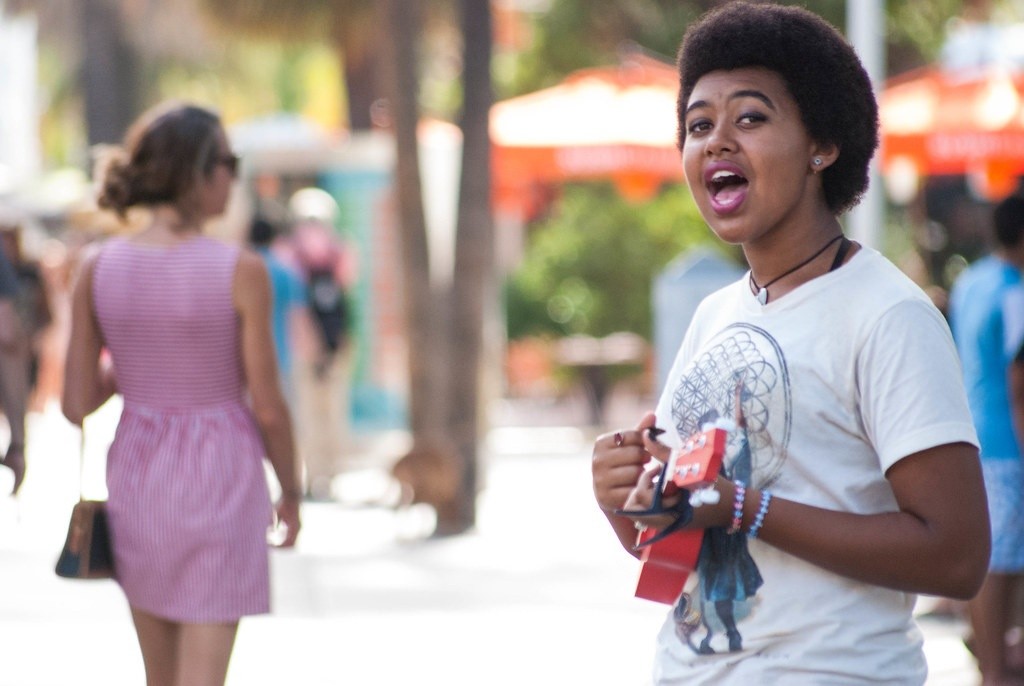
[{"x1": 217, "y1": 155, "x2": 238, "y2": 177}]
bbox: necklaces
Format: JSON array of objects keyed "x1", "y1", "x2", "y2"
[{"x1": 749, "y1": 234, "x2": 844, "y2": 306}]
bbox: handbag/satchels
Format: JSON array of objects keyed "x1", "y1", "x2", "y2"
[{"x1": 57, "y1": 500, "x2": 116, "y2": 578}]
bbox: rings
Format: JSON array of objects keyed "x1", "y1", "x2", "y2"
[{"x1": 615, "y1": 431, "x2": 624, "y2": 447}]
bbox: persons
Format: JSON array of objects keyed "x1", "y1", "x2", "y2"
[
  {"x1": 592, "y1": 3, "x2": 992, "y2": 686},
  {"x1": 1, "y1": 106, "x2": 356, "y2": 686},
  {"x1": 948, "y1": 175, "x2": 1023, "y2": 685}
]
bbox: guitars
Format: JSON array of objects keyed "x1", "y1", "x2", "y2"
[{"x1": 634, "y1": 427, "x2": 727, "y2": 606}]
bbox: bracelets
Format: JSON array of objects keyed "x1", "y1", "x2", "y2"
[
  {"x1": 745, "y1": 491, "x2": 773, "y2": 539},
  {"x1": 725, "y1": 479, "x2": 745, "y2": 535}
]
